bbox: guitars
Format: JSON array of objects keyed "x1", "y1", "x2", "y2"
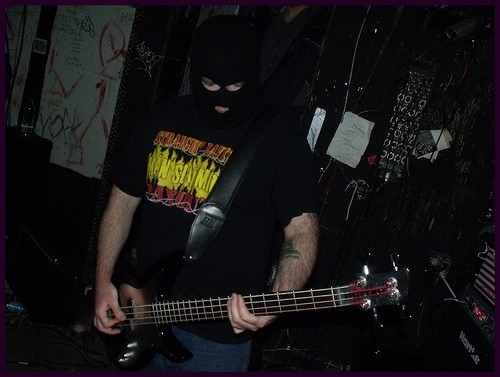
[{"x1": 67, "y1": 247, "x2": 415, "y2": 372}]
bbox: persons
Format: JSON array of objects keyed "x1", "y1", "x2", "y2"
[
  {"x1": 252, "y1": 5, "x2": 331, "y2": 125},
  {"x1": 91, "y1": 14, "x2": 321, "y2": 372}
]
